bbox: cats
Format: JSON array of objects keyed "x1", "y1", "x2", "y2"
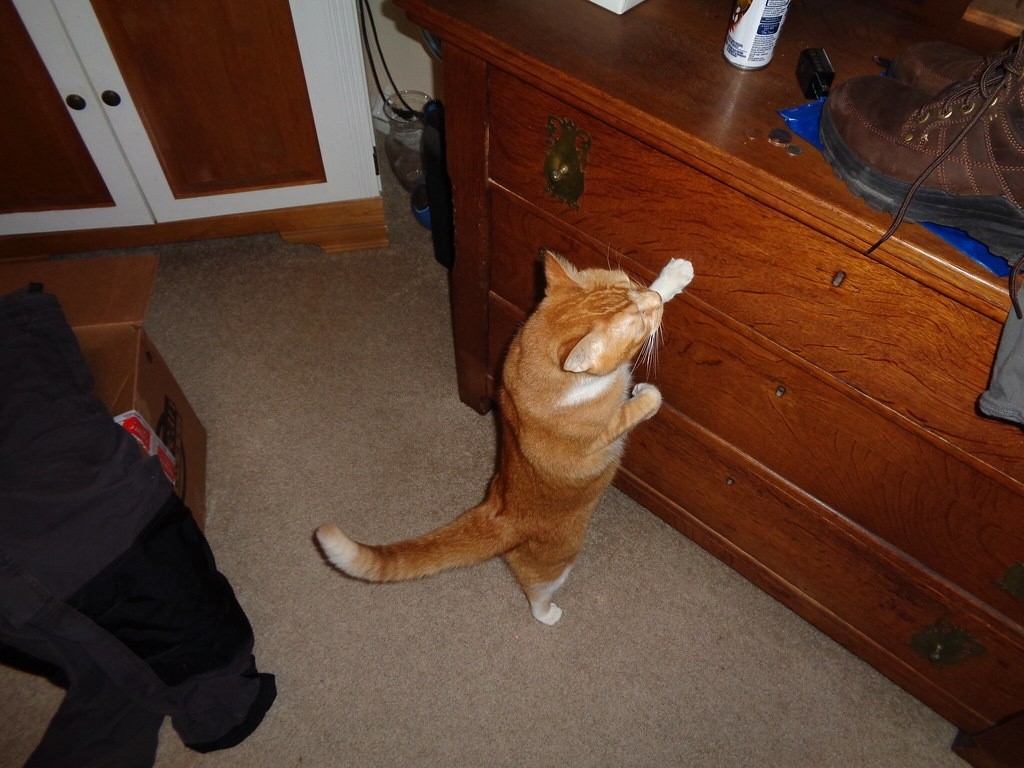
[{"x1": 313, "y1": 241, "x2": 696, "y2": 627}]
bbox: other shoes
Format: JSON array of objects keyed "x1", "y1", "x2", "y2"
[
  {"x1": 888, "y1": 31, "x2": 1024, "y2": 97},
  {"x1": 820, "y1": 42, "x2": 1024, "y2": 320}
]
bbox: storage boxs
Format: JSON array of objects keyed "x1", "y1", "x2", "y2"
[{"x1": 0, "y1": 255, "x2": 217, "y2": 541}]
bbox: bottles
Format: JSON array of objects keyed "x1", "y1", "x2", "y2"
[
  {"x1": 383, "y1": 89, "x2": 434, "y2": 193},
  {"x1": 722, "y1": 0, "x2": 792, "y2": 71}
]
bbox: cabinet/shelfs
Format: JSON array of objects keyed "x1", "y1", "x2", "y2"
[
  {"x1": 382, "y1": 0, "x2": 1024, "y2": 768},
  {"x1": 0, "y1": 0, "x2": 389, "y2": 252}
]
